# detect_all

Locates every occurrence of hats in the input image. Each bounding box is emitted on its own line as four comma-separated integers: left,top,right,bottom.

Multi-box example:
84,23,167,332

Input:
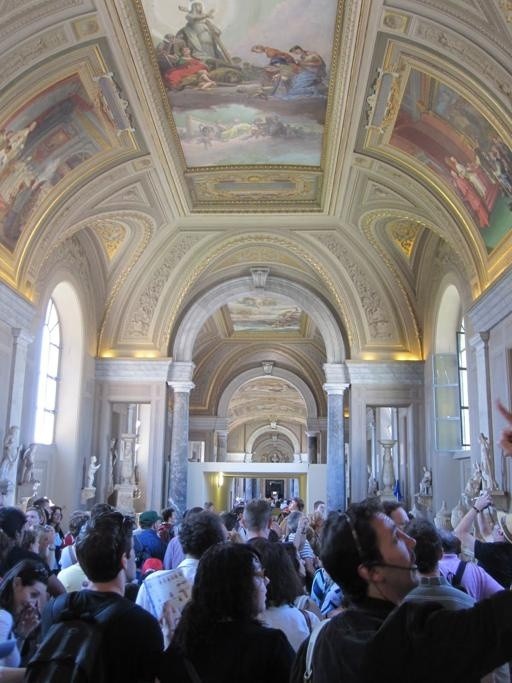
6,547,51,585
139,511,161,525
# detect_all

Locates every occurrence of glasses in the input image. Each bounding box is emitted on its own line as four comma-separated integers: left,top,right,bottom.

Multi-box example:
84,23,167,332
244,567,266,579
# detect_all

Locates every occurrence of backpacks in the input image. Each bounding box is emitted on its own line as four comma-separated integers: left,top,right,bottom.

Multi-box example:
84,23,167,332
23,591,129,683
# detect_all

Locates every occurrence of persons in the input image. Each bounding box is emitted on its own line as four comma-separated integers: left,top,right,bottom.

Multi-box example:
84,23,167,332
465,432,499,492
2,493,512,683
0,119,39,166
122,442,135,484
446,134,511,225
21,443,37,484
419,465,432,496
158,2,327,98
0,425,22,494
88,456,101,488
